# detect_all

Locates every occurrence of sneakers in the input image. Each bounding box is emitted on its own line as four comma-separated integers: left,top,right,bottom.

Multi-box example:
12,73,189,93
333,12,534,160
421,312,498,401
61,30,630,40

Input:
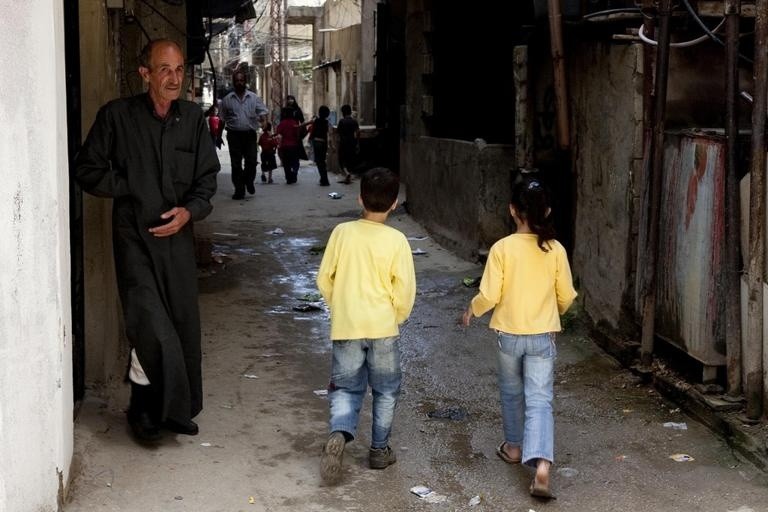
269,178,273,183
287,172,297,183
345,174,352,184
320,433,345,485
262,175,267,181
320,178,330,185
369,444,397,468
245,177,255,194
232,192,244,200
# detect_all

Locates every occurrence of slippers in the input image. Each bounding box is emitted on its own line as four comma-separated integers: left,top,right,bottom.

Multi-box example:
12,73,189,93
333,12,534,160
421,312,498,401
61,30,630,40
530,478,551,497
497,441,522,463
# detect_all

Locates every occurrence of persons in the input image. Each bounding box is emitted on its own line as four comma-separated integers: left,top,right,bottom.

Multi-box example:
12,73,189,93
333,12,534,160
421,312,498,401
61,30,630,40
208,106,220,147
280,95,308,168
276,108,304,185
70,37,220,446
461,177,579,500
293,105,333,187
329,105,362,184
314,165,416,487
214,71,270,201
258,123,279,185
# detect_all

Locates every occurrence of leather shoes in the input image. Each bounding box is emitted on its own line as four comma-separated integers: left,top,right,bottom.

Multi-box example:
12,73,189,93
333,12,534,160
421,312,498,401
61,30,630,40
169,418,198,435
128,407,166,444
